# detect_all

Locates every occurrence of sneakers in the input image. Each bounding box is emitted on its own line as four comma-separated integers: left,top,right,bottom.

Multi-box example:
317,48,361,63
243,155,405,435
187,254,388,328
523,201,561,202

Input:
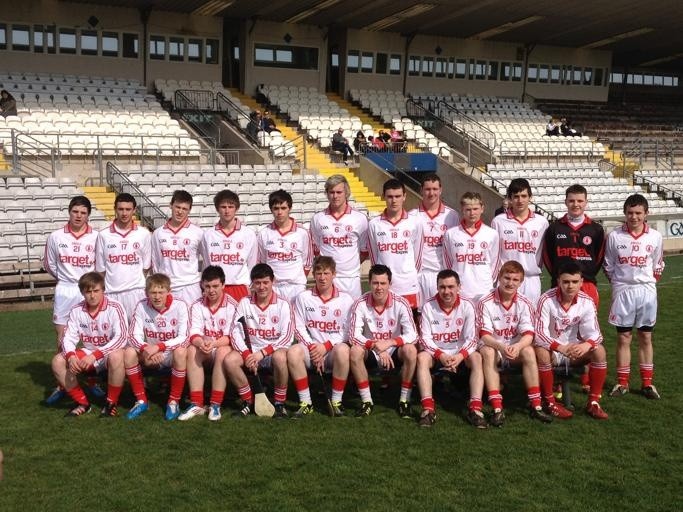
354,402,374,417
274,403,287,420
396,401,416,419
608,384,631,397
231,402,253,418
126,403,149,419
166,404,180,421
333,401,347,418
641,385,662,401
291,403,314,419
177,403,222,422
45,383,119,418
419,378,611,430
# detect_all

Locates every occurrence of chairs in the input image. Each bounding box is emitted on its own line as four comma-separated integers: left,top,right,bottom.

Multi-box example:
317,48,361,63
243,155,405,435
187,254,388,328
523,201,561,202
0,69,683,303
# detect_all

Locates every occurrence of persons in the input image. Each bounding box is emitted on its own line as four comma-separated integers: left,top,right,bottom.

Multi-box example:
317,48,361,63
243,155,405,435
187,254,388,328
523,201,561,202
310,173,371,306
50,271,129,421
152,190,205,301
244,106,410,164
601,194,665,399
532,257,612,420
344,264,420,423
95,194,155,323
416,268,490,429
561,117,584,138
538,183,606,399
440,191,503,304
0,91,18,118
178,265,240,423
494,198,510,216
489,178,550,312
123,273,190,421
200,189,258,296
286,256,353,420
406,171,462,310
544,116,560,137
43,194,108,407
367,177,422,323
478,258,553,427
223,263,295,420
255,189,314,300
